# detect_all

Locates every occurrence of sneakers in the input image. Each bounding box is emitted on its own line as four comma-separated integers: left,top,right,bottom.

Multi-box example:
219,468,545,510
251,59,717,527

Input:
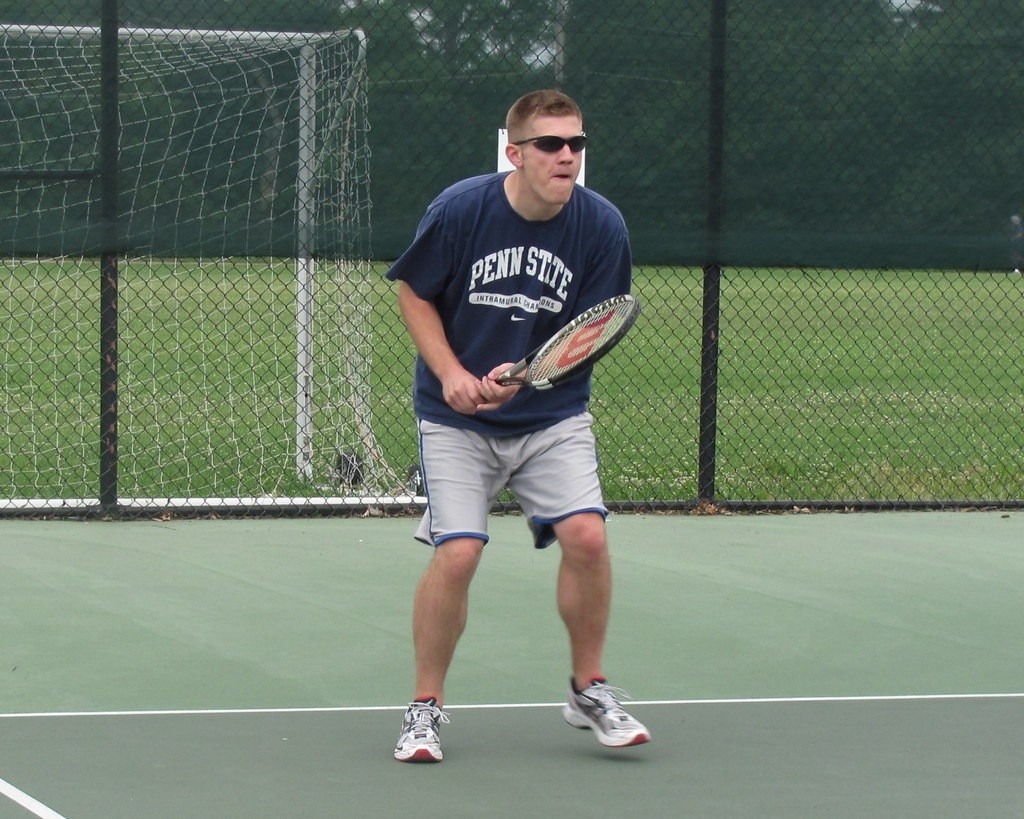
561,672,652,748
393,696,451,764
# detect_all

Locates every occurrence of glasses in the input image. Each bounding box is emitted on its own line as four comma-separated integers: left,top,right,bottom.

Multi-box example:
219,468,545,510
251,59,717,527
513,134,588,152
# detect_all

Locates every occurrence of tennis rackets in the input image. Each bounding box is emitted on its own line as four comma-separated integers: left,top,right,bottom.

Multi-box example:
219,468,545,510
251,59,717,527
468,293,640,393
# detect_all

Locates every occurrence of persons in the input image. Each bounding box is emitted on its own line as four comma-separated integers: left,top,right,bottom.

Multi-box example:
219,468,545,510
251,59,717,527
386,88,652,763
